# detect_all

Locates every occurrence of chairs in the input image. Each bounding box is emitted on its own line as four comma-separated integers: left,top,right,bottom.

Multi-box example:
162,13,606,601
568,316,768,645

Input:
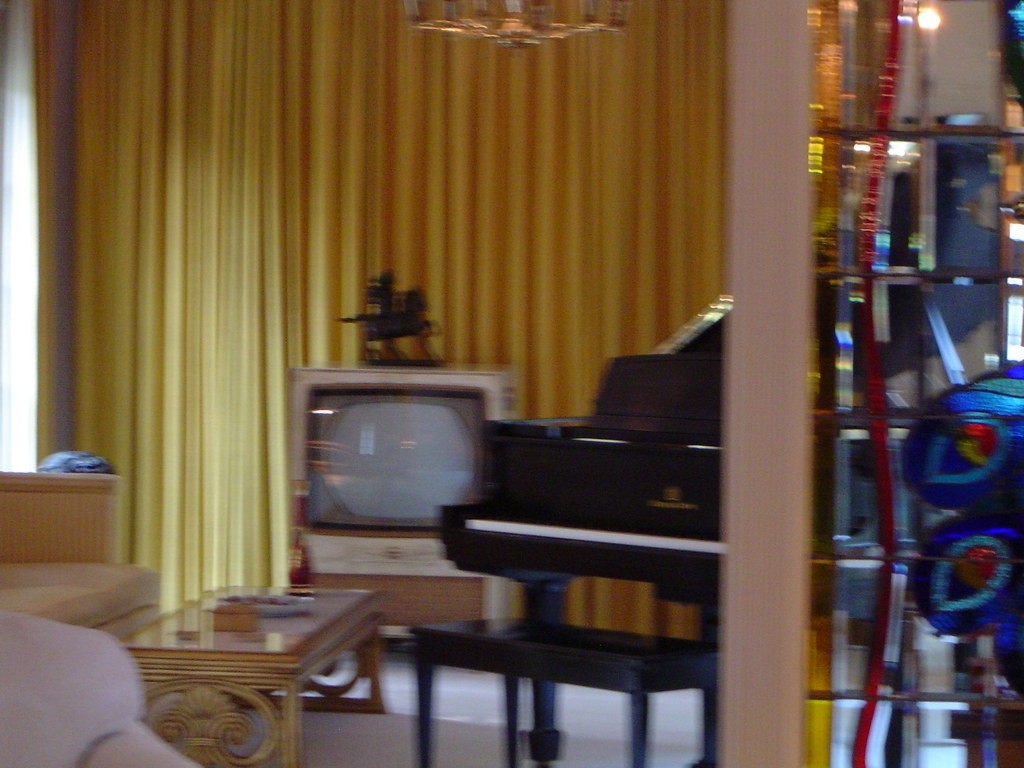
0,610,200,768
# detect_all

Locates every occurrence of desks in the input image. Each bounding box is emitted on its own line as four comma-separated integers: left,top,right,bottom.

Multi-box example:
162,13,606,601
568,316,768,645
113,589,386,768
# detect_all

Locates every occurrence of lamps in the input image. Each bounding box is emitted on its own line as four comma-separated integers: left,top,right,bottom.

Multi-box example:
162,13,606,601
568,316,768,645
404,0,631,50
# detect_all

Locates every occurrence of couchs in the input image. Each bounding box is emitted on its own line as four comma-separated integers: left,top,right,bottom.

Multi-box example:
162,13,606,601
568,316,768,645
0,471,159,637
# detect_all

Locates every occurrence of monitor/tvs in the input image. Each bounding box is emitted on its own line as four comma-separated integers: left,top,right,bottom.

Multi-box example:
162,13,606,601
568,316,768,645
285,366,512,578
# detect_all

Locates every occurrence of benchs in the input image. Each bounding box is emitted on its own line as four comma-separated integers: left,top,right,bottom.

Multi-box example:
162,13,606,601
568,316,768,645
405,616,716,768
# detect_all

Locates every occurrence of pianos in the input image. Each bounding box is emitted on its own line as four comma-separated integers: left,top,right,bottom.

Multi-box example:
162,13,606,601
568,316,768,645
436,350,721,768
